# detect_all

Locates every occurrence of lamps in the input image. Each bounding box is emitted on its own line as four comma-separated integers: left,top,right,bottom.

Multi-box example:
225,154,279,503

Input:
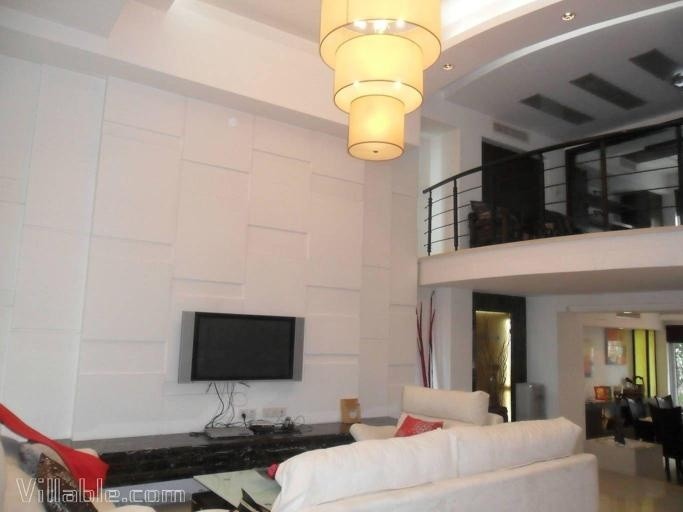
318,0,443,160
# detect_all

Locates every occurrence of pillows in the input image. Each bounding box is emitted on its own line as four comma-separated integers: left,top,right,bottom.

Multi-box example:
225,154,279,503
34,451,98,512
18,438,68,475
394,413,444,437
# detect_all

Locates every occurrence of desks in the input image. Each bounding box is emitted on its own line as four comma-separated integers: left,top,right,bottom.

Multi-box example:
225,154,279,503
76,416,400,490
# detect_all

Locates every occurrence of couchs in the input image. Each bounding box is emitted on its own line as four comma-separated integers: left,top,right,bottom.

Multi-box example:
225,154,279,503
192,414,602,512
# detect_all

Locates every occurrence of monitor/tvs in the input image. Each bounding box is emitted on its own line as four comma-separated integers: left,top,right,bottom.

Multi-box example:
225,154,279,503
176,311,305,384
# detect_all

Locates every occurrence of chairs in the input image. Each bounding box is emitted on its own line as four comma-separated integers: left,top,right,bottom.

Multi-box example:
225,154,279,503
624,395,683,482
1,400,162,512
346,383,503,442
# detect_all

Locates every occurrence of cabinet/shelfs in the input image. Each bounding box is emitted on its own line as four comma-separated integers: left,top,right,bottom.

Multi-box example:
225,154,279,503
586,400,617,439
